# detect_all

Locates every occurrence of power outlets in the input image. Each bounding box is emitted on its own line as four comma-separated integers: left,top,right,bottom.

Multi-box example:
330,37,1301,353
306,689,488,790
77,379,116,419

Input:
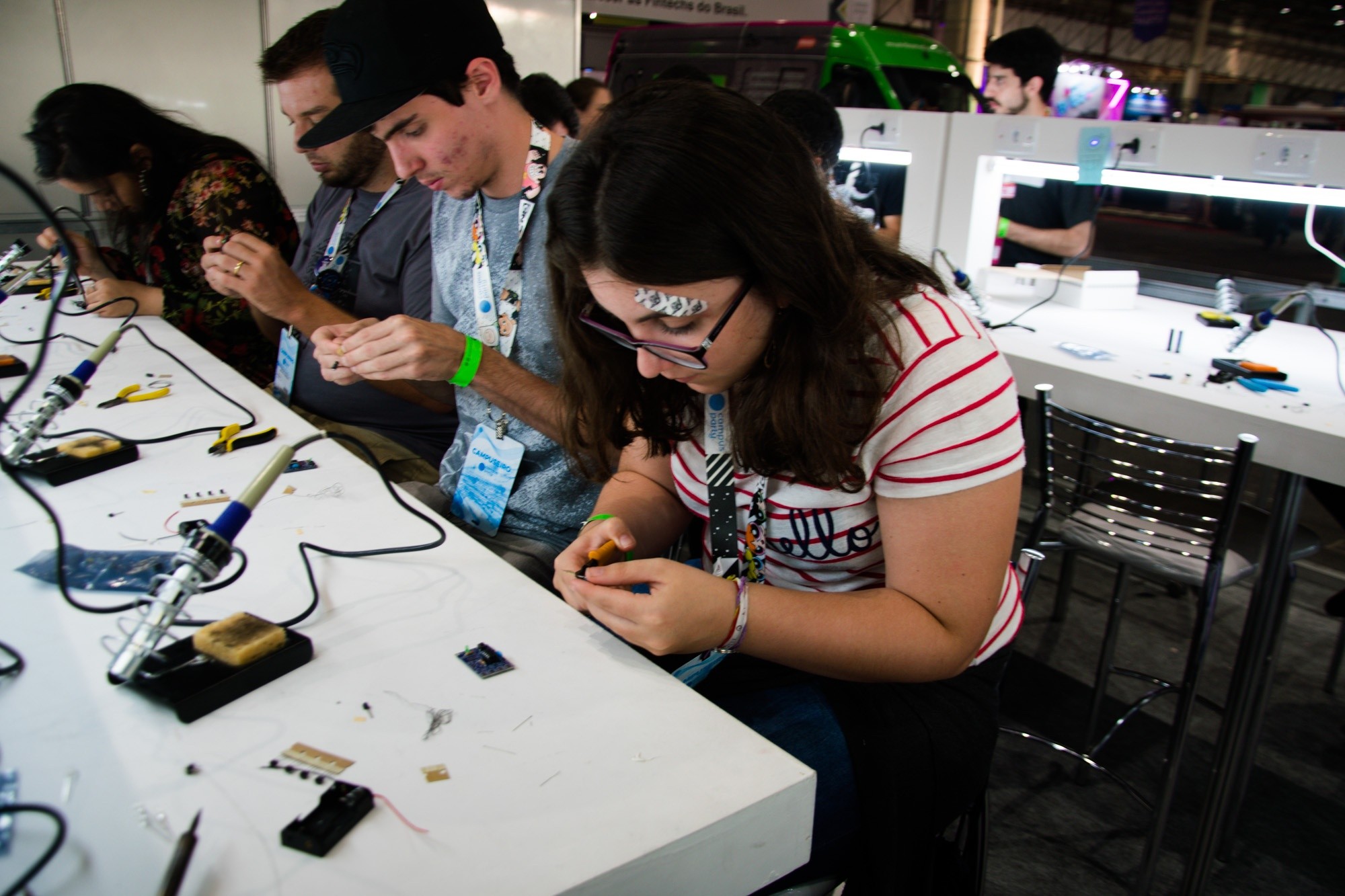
1111,129,1159,166
869,119,895,145
1255,132,1317,175
993,122,1036,154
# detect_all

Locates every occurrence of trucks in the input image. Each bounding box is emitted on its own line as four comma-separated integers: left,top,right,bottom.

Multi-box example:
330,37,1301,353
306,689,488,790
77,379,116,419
601,16,997,114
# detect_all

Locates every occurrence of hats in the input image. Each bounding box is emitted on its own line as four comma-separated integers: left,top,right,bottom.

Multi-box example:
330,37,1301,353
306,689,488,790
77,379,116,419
296,0,504,149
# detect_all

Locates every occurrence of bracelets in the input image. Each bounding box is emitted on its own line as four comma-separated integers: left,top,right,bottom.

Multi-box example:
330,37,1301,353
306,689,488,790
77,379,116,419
713,575,750,654
577,514,635,562
997,217,1009,239
447,333,483,390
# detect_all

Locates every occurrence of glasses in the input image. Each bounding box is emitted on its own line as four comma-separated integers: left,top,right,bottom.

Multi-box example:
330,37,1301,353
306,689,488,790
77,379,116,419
575,277,754,370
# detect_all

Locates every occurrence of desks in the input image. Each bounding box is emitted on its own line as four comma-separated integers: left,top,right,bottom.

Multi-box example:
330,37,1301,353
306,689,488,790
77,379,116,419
934,273,1345,798
0,256,817,896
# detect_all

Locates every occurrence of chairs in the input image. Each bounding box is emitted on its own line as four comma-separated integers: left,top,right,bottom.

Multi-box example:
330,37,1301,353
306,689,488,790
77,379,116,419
606,322,1343,896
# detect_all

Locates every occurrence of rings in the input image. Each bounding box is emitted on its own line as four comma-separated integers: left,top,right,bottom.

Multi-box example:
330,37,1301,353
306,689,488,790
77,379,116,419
234,261,245,276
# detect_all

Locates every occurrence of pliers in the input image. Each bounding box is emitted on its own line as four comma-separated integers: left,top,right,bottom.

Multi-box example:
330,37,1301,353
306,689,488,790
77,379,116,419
34,283,79,300
97,384,170,409
1234,375,1299,393
208,423,278,454
574,540,617,580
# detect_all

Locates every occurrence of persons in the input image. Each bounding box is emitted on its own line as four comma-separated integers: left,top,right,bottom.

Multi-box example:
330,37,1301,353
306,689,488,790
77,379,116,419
823,70,902,239
21,83,301,389
523,74,612,141
1303,476,1345,617
983,24,1099,267
546,81,1028,895
759,89,844,186
296,0,628,589
201,10,459,484
